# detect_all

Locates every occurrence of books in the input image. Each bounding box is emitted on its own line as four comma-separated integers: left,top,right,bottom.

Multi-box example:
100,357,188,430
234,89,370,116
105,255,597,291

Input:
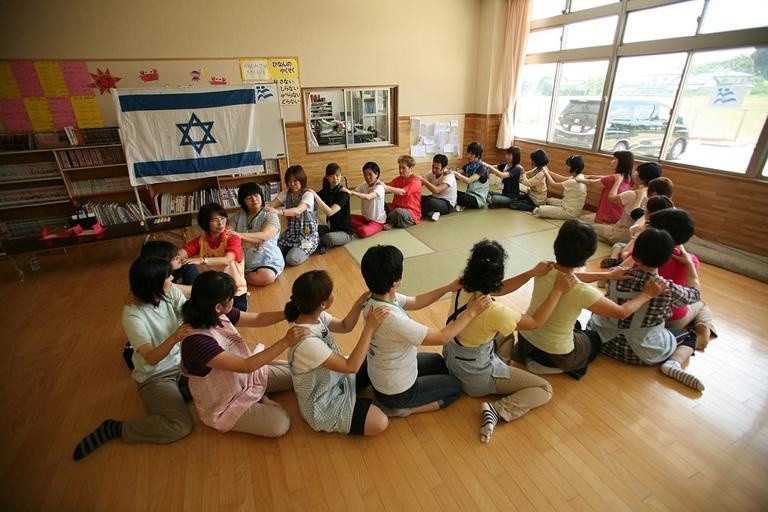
78,200,153,226
0,126,122,152
0,162,62,181
0,213,76,238
220,187,241,208
57,147,127,169
71,177,135,195
257,182,281,203
232,159,278,178
154,192,222,215
0,185,70,206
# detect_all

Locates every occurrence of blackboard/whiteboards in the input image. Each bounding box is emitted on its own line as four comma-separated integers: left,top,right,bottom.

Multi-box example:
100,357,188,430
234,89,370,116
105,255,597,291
1,56,304,130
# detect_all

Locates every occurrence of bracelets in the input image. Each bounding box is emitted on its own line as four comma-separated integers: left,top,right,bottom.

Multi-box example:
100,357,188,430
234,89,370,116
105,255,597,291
281,209,285,216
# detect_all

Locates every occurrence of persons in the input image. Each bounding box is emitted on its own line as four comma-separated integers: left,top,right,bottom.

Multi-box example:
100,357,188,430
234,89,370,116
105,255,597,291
630,196,673,236
479,146,522,207
446,142,488,212
303,163,354,254
261,164,321,265
511,219,669,381
73,256,194,461
586,228,705,391
621,209,717,351
443,239,577,443
575,152,639,228
123,240,202,368
338,162,388,238
179,202,248,310
378,155,428,230
532,155,587,219
591,162,661,246
509,149,549,212
226,182,285,285
180,270,312,437
601,177,680,268
362,245,492,417
415,154,458,221
284,272,391,437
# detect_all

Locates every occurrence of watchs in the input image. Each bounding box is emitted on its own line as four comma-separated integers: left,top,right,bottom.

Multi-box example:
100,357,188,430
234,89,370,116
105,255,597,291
202,257,207,264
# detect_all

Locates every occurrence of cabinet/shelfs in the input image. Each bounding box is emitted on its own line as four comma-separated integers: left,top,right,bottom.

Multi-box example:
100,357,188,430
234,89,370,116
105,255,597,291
0,143,288,254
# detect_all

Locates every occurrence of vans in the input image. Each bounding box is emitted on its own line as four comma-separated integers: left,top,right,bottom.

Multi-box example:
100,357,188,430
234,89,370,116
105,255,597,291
554,99,688,160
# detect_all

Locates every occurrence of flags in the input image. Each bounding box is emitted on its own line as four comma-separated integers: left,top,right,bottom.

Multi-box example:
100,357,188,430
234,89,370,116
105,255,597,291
110,85,265,186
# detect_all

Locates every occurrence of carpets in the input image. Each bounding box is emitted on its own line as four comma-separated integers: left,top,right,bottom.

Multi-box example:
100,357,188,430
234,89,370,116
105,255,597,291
316,175,613,330
